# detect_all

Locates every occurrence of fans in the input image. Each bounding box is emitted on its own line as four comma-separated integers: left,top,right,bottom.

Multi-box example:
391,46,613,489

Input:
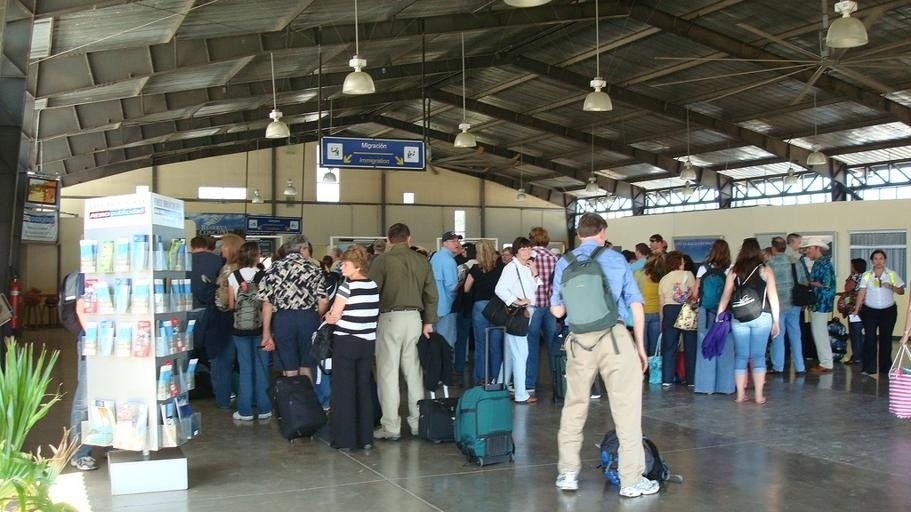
645,1,911,107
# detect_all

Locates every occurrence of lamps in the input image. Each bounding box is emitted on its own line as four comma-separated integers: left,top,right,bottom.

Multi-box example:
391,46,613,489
805,85,827,166
453,32,477,147
582,1,613,112
265,52,291,139
824,0,869,49
783,160,798,185
252,146,600,204
341,1,375,94
682,178,694,195
679,103,698,182
503,1,551,8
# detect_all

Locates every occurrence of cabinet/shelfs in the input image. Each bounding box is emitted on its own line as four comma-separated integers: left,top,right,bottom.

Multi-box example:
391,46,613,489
77,184,207,497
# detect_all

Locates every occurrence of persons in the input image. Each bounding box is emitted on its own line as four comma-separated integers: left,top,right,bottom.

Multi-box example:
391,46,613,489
187,229,388,453
843,258,866,365
618,232,836,404
899,307,910,345
851,250,905,375
58,270,118,471
549,214,659,497
411,227,603,404
366,222,439,440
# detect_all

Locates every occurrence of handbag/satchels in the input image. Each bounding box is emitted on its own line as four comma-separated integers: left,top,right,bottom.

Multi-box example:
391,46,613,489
310,324,333,361
888,344,911,418
674,301,699,331
649,333,663,384
837,290,856,313
482,297,529,336
792,283,818,306
827,318,847,361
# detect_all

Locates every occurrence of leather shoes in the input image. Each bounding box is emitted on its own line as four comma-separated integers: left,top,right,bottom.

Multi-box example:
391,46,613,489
768,365,833,376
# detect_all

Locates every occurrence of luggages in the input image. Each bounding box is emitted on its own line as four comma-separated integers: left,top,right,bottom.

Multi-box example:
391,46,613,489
454,326,513,466
257,345,328,443
419,398,456,443
553,355,566,404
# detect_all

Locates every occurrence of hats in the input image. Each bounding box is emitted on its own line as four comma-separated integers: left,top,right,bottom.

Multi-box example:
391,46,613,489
442,232,463,242
799,237,830,251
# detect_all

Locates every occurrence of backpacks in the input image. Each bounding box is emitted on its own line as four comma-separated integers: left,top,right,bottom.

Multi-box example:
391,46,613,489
560,247,618,334
233,269,266,336
57,272,82,335
600,430,671,486
731,264,767,322
699,263,729,312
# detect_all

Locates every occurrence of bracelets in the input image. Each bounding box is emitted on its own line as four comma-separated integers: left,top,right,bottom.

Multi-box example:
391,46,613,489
809,282,811,286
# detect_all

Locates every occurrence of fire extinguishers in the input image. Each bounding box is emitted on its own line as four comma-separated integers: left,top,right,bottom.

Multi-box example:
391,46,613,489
8,266,22,329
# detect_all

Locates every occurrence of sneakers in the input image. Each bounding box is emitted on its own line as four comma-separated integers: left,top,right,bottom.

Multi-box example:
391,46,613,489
556,471,579,490
232,412,254,421
619,478,661,497
412,430,419,437
373,427,401,441
509,382,538,403
103,450,107,458
258,412,272,419
71,455,98,470
